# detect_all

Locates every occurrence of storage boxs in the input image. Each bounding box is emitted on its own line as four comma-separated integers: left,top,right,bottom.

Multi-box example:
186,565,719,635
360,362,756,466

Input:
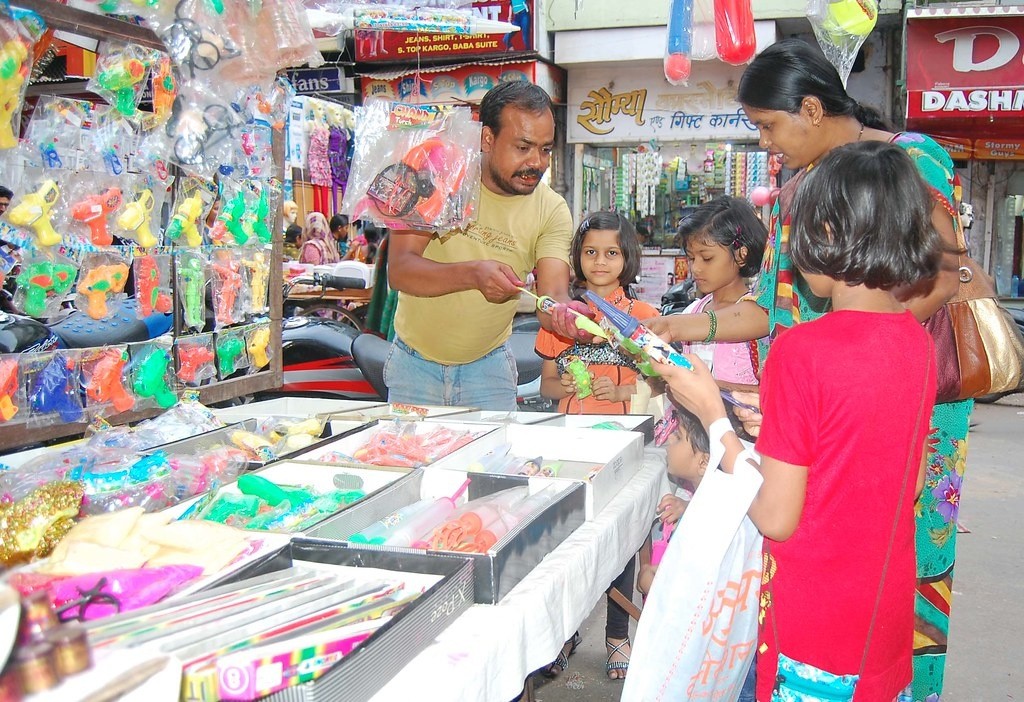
282,261,375,289
0,395,654,701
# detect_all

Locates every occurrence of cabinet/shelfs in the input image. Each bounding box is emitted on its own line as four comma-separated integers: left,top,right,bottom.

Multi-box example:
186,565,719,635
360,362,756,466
582,146,769,249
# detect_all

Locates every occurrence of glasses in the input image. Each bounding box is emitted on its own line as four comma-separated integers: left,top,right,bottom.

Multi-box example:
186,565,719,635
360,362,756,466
175,104,244,166
163,17,220,81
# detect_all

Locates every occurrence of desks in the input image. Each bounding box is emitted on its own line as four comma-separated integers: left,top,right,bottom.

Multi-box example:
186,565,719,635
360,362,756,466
360,443,669,702
283,286,374,321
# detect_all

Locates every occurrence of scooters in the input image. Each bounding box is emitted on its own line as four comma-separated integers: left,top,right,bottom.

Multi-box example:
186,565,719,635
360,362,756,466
660,278,702,316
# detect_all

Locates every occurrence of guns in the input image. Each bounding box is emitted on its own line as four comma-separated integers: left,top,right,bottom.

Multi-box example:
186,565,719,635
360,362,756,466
0,31,286,427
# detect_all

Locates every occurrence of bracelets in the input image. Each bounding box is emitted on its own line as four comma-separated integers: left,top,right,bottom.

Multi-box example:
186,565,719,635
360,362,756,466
702,310,716,344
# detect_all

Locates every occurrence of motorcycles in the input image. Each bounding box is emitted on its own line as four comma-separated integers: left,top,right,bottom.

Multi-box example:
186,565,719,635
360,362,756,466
209,315,553,401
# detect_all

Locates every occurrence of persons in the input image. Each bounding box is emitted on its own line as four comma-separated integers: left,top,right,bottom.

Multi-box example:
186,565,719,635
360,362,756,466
382,80,596,411
533,38,974,702
283,200,391,318
307,99,360,218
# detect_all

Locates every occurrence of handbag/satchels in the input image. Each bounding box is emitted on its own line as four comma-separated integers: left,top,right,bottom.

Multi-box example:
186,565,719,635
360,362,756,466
619,416,765,702
888,131,1024,405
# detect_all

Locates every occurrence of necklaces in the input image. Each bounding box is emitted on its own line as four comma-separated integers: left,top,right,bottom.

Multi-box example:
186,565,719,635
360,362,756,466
858,122,864,141
627,299,635,316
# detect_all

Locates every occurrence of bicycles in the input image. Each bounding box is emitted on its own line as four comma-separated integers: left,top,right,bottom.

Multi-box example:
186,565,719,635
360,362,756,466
283,273,365,330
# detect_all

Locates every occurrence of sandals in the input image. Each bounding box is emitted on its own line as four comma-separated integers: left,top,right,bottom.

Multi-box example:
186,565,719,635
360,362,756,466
605,635,631,679
541,630,582,679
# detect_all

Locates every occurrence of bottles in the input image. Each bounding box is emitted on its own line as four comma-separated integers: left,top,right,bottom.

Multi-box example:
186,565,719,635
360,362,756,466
1010,275,1019,298
